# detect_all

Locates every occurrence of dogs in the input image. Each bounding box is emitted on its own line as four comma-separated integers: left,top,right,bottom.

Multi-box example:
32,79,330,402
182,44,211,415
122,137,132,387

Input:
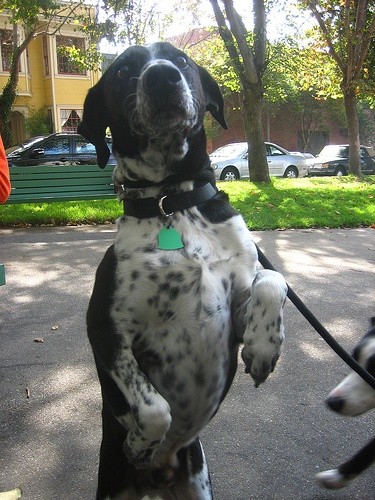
76,41,288,500
313,315,374,491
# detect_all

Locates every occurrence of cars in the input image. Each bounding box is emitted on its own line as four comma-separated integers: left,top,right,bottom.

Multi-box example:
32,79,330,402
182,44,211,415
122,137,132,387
5,132,117,167
303,153,317,168
208,141,307,181
305,144,375,176
